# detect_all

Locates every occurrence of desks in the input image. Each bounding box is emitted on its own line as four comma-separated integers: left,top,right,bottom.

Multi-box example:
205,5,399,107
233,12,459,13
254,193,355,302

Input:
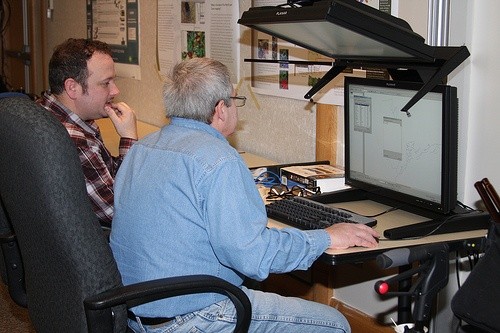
95,118,488,333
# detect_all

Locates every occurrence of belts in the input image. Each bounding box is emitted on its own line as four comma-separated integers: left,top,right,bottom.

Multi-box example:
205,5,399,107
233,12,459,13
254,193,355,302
127,310,175,326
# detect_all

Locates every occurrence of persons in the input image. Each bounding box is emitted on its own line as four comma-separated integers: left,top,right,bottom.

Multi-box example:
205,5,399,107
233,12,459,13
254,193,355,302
34,37,137,223
113,57,380,333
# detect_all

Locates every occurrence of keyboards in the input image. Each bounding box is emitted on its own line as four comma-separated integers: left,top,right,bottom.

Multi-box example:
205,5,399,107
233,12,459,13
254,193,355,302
264,196,377,231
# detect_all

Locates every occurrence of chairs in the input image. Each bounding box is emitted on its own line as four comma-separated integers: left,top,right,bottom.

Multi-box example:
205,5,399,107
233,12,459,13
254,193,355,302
0,93,252,333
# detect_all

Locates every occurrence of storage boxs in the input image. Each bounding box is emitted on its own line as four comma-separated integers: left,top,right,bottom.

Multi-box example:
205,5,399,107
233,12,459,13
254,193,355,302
281,164,351,194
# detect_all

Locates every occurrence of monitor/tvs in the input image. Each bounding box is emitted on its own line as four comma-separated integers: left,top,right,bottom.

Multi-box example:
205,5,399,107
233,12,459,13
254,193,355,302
342,76,458,214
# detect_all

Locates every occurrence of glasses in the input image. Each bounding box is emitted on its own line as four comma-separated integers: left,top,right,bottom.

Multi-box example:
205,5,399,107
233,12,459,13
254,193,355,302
266,183,321,199
215,96,247,108
